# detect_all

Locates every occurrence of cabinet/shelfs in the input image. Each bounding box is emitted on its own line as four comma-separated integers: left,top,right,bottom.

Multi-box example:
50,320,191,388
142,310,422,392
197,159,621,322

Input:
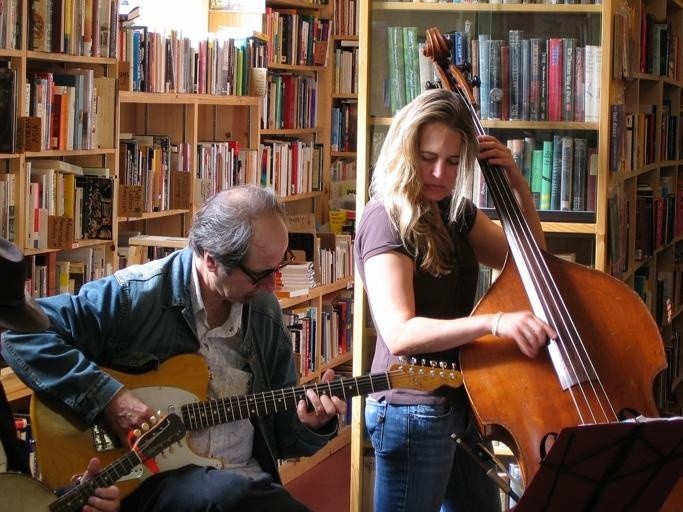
359,0,681,465
0,1,359,501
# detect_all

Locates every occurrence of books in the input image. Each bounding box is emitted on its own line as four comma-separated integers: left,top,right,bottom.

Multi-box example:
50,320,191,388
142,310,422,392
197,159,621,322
461,129,597,212
261,69,318,130
1,2,119,58
197,140,258,201
607,178,682,413
386,26,601,124
24,242,111,298
330,104,356,193
334,2,359,95
274,208,353,298
265,8,331,68
118,131,190,213
613,2,681,174
282,289,352,377
260,136,324,198
1,60,121,154
1,157,114,253
118,230,178,271
117,4,267,96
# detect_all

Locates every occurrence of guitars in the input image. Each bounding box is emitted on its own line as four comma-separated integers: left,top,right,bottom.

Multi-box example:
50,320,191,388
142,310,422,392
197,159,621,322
31,353,464,500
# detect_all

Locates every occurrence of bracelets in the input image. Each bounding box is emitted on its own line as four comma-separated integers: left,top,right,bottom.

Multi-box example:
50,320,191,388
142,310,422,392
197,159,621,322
491,310,504,337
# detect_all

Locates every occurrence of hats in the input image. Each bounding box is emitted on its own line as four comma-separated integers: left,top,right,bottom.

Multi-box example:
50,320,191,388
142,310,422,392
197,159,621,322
0,234,52,333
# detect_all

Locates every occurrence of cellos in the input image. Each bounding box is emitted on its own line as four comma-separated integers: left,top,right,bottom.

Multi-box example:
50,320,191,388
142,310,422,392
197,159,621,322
420,25,683,510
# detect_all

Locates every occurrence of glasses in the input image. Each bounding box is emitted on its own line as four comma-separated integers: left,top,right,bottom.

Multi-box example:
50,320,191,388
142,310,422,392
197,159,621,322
240,249,295,285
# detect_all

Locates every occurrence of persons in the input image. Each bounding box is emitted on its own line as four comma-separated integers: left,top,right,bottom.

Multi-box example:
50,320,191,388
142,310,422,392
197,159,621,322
0,179,346,511
354,89,556,511
74,456,122,510
